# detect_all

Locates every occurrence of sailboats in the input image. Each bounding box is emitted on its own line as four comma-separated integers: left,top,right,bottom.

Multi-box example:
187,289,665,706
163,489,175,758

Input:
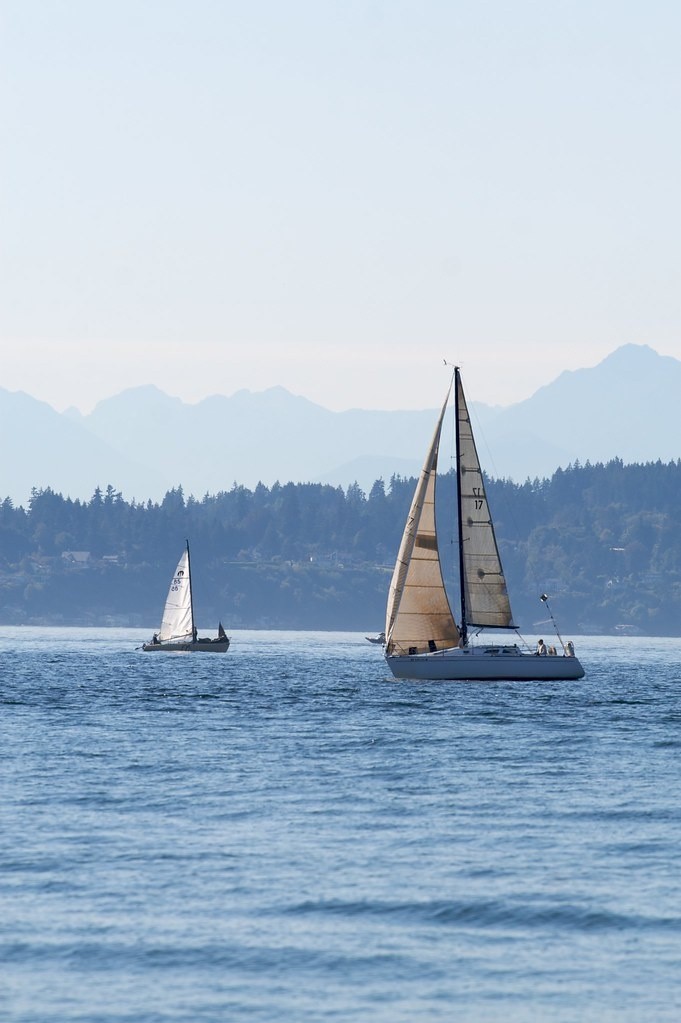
141,541,231,653
379,366,587,680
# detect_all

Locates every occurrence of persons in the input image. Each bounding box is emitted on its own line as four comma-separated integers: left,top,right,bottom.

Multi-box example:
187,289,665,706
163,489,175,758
457,621,468,645
153,634,160,644
534,638,575,657
